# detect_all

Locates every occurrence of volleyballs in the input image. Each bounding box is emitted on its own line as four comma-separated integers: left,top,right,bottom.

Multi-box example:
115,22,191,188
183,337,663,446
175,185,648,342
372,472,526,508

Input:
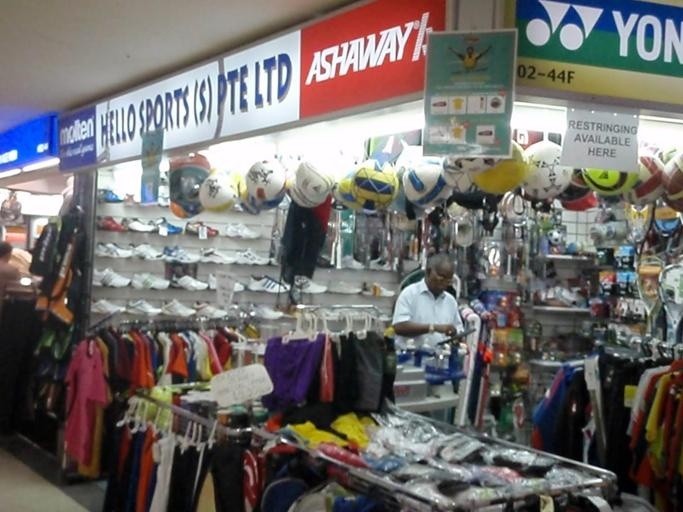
200,140,683,214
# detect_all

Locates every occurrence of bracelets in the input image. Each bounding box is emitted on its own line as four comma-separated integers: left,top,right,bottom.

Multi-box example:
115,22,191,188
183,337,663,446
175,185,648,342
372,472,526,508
428,323,434,333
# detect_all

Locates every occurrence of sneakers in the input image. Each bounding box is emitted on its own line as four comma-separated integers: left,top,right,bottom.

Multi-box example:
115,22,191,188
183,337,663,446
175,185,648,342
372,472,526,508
327,219,353,235
99,189,126,205
95,268,395,322
95,215,261,239
316,251,394,271
96,242,278,265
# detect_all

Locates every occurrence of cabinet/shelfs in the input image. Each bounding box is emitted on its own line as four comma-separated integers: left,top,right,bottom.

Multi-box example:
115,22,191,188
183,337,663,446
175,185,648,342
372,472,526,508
530,252,598,369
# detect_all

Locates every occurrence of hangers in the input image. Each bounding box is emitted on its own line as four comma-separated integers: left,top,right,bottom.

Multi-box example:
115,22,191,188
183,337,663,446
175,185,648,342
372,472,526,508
114,389,223,458
281,303,387,344
83,315,258,355
604,332,683,380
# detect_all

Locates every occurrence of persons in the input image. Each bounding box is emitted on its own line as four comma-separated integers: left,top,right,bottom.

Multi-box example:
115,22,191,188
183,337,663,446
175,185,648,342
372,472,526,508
0,240,23,302
390,251,466,352
